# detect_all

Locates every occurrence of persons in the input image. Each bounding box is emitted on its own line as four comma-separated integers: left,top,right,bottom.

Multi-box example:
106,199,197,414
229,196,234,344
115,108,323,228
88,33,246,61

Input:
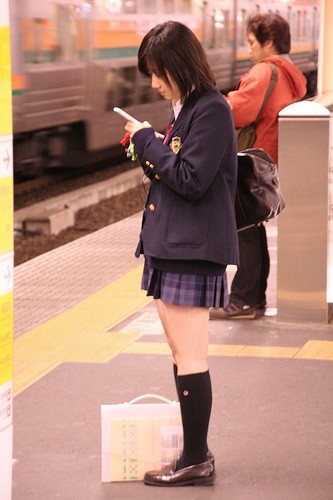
209,14,307,322
125,21,237,488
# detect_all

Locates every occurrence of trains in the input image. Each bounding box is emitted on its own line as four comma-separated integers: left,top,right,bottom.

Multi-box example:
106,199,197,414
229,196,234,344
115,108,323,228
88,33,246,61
10,0,323,179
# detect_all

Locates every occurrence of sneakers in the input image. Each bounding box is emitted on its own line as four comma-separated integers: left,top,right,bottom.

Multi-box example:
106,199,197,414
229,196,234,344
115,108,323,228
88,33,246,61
209,295,266,319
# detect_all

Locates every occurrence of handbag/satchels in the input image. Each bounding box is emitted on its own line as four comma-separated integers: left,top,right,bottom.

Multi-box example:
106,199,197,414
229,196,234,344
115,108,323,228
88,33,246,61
235,60,277,152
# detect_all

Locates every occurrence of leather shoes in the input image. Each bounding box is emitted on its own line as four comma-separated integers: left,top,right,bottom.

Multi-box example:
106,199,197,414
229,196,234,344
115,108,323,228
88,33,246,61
144,450,216,487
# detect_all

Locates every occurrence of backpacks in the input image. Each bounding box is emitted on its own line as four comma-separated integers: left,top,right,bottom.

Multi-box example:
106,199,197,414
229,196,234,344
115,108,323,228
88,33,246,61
236,148,285,233
